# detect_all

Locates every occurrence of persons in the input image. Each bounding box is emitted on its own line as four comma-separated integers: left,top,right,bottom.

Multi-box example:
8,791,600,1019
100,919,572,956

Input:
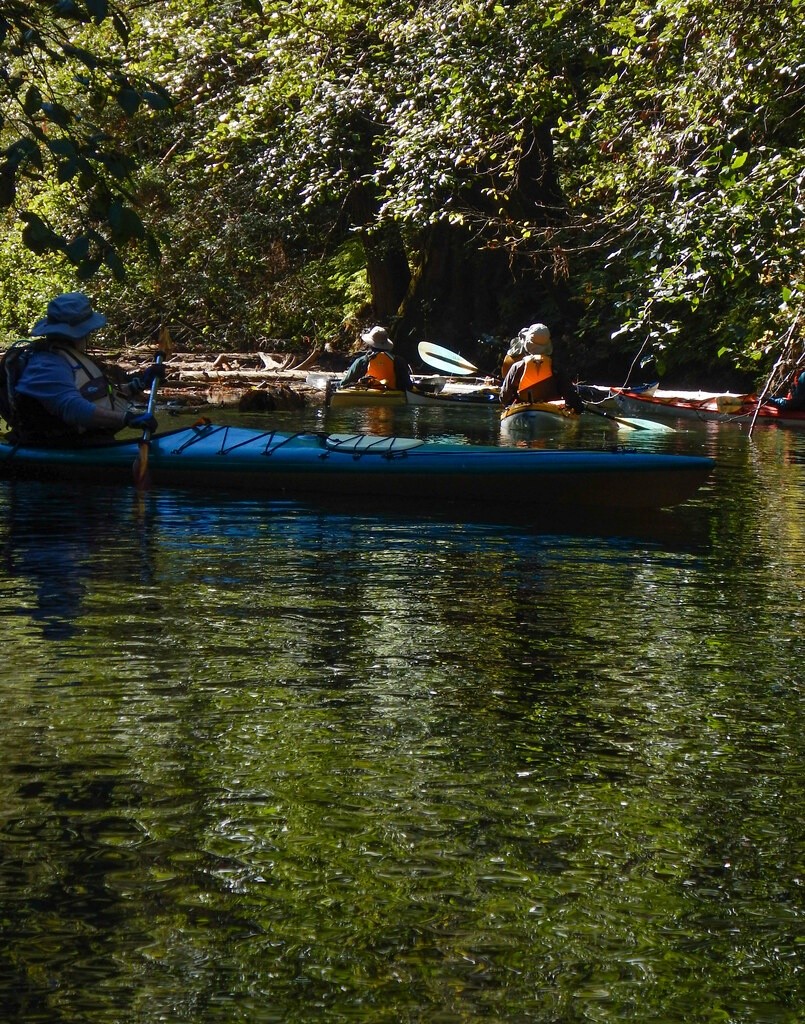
17,292,165,448
335,326,413,392
498,322,584,414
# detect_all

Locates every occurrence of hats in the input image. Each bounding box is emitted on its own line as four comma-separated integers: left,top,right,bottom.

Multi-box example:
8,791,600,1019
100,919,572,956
30,293,106,338
360,325,394,349
517,322,553,356
507,337,523,356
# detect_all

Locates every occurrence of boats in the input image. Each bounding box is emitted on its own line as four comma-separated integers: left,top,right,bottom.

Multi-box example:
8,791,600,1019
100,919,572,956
407,374,505,403
0,419,719,516
609,385,805,427
500,402,581,444
316,383,407,407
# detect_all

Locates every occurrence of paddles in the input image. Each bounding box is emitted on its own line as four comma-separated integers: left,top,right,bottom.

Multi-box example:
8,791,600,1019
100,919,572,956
415,336,670,433
134,324,173,484
305,372,446,394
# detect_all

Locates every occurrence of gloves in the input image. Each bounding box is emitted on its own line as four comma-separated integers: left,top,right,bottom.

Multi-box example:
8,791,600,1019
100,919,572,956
122,411,158,433
132,364,167,392
